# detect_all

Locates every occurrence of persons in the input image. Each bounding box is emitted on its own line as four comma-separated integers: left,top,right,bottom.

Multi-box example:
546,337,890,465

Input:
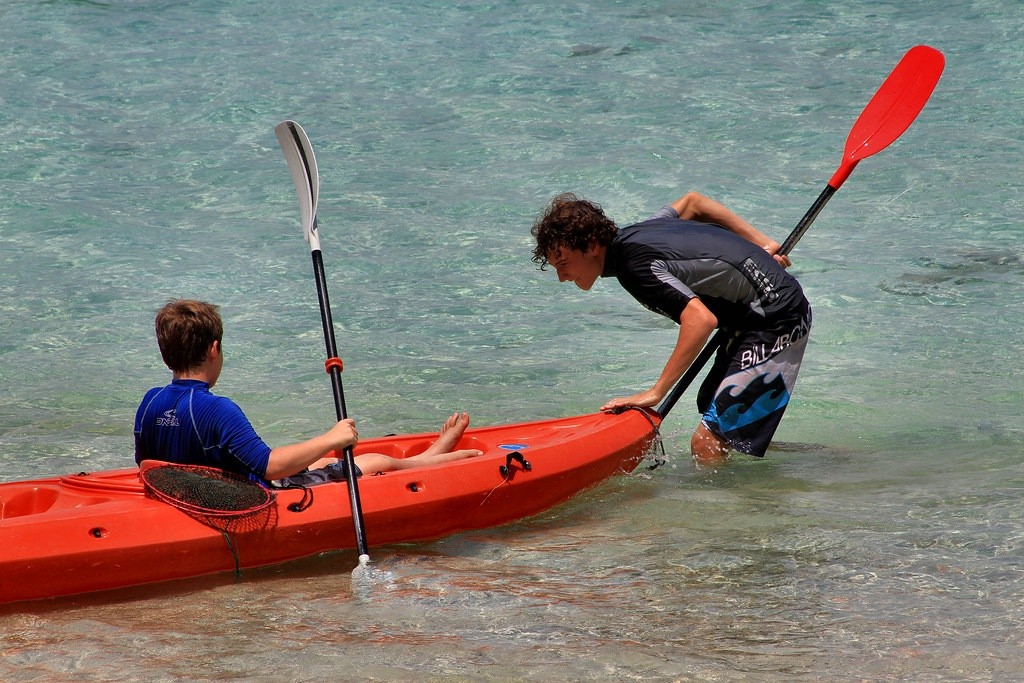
531,191,813,470
134,296,483,489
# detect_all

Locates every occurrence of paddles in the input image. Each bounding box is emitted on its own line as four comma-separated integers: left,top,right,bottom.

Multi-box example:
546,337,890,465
274,119,376,574
656,44,947,422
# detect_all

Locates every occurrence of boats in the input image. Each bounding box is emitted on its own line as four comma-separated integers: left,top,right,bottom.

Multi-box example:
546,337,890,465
1,404,664,610
